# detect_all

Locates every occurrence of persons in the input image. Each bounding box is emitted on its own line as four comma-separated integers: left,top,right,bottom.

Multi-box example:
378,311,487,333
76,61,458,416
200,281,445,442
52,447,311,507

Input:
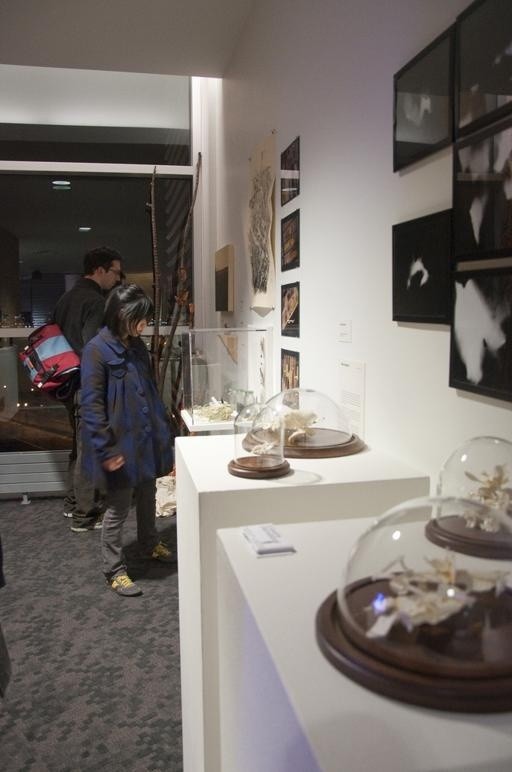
51,247,125,532
81,283,179,597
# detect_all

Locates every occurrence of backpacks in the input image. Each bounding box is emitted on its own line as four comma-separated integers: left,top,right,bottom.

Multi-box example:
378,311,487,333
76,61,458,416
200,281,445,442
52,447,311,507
17,296,77,402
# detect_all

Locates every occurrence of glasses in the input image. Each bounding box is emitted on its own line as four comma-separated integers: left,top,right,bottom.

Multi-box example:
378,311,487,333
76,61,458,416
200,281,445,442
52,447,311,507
107,268,121,275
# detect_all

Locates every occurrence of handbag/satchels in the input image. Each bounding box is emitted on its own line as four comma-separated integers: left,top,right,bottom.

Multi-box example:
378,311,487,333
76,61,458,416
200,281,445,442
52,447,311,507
17,320,81,402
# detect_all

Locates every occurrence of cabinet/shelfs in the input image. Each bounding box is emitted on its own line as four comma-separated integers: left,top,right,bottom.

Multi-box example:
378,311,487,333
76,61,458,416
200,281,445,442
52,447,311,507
173,430,431,772
218,517,512,772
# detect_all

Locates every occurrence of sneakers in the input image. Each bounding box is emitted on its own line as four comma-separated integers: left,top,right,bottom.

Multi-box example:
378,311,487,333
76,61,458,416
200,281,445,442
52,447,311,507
106,569,142,598
142,541,177,563
61,507,79,516
68,518,107,531
